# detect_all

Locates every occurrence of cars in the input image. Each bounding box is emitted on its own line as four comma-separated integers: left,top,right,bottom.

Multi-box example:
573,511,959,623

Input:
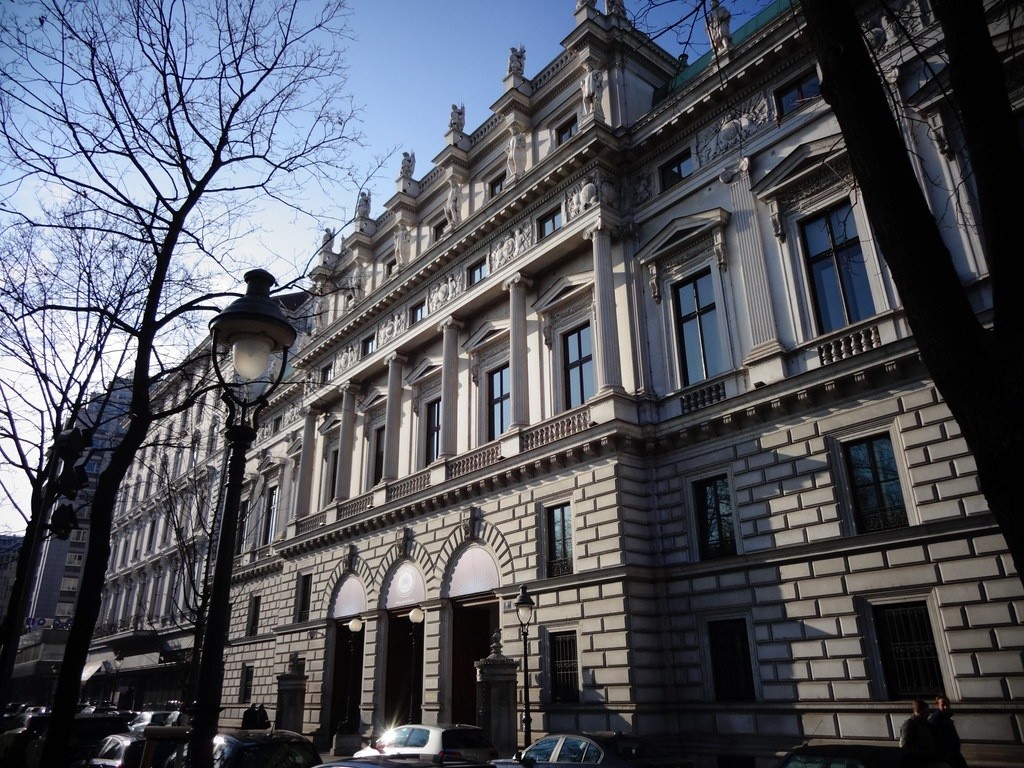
2,700,968,768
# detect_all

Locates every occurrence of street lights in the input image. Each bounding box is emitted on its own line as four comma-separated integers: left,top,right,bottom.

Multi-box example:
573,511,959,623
337,619,363,734
187,269,299,766
409,607,425,724
515,583,535,747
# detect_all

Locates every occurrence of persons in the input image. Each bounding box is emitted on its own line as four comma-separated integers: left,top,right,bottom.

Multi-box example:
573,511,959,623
357,190,371,219
400,148,416,177
898,696,969,768
503,122,527,177
579,63,603,111
448,99,465,132
443,177,464,225
321,227,336,250
351,260,363,298
241,702,272,730
705,0,731,53
394,222,411,265
508,44,527,76
